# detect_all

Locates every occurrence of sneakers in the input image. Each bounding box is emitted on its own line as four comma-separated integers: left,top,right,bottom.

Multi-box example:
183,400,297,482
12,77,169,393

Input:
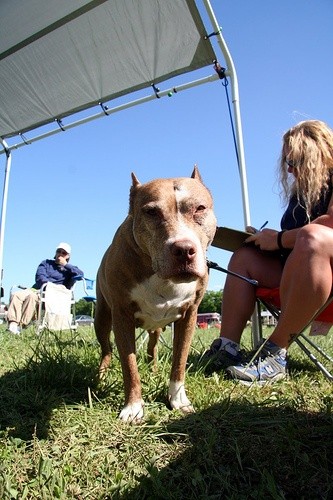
200,338,239,372
226,338,287,382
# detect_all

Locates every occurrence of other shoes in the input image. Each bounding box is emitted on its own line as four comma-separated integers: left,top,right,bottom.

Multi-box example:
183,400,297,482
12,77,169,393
7,323,19,335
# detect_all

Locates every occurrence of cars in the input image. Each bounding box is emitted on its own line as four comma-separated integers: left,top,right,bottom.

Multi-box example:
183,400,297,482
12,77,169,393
72,315,96,325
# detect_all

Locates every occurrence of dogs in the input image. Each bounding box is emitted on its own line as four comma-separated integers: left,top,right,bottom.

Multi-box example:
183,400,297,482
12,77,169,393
91,164,217,423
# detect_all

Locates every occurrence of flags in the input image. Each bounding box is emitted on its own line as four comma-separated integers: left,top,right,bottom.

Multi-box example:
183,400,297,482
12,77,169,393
84,278,93,290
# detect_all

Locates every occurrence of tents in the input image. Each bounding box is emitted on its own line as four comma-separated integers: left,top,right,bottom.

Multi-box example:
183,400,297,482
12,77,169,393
0,0,261,350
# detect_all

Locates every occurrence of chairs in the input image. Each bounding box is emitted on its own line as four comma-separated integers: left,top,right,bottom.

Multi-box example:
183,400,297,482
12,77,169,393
10,277,96,337
255,288,333,380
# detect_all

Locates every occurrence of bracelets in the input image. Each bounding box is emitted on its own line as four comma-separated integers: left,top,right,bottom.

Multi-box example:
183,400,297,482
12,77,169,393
277,229,286,249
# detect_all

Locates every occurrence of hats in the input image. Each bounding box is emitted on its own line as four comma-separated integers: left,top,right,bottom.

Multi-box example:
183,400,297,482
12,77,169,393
57,243,71,253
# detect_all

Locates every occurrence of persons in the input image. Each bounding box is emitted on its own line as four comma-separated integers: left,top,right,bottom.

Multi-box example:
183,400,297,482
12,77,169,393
6,241,84,334
190,123,333,385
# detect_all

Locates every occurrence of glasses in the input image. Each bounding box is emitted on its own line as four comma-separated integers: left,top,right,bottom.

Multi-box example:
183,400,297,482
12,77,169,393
285,158,305,169
57,250,67,255
